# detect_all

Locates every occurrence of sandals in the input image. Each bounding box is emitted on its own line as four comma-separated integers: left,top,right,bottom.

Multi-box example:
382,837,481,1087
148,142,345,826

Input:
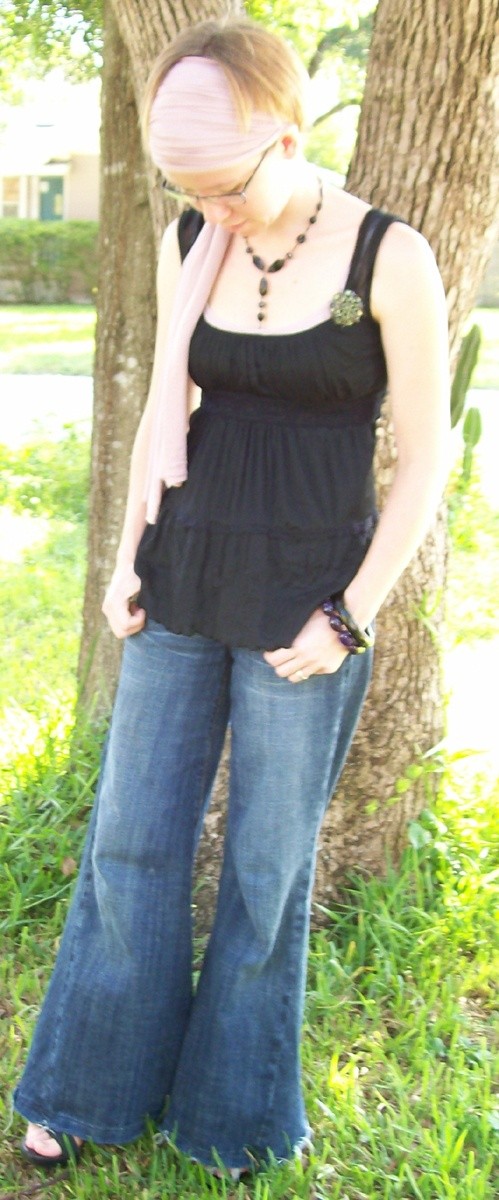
21,1120,89,1161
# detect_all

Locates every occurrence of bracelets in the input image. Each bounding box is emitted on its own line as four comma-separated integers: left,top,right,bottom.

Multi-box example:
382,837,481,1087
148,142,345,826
321,590,376,654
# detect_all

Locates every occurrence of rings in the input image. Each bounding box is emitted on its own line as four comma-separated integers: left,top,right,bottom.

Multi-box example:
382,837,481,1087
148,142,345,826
296,673,308,681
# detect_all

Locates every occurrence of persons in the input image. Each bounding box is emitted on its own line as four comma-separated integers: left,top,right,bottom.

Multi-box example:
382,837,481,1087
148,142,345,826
10,20,453,1193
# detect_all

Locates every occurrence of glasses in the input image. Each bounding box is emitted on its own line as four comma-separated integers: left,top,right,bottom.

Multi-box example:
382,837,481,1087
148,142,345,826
160,138,274,206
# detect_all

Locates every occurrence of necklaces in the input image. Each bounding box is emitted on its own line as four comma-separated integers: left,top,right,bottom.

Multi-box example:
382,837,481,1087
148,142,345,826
241,177,324,321
